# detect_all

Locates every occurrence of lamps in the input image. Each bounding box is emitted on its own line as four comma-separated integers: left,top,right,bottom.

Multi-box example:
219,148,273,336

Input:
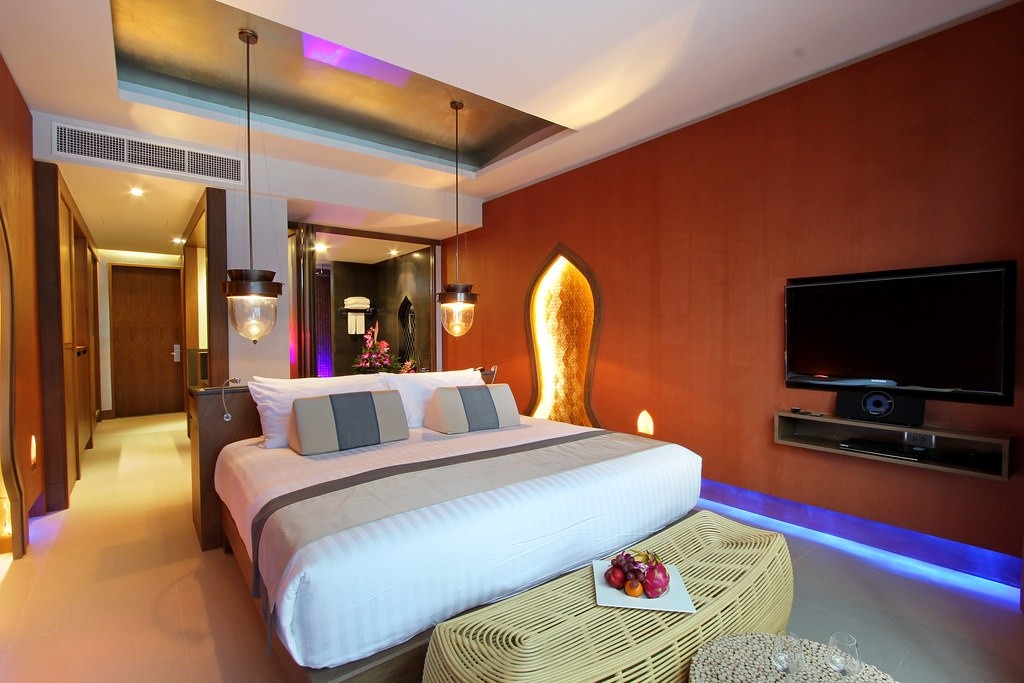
437,100,479,339
223,29,284,345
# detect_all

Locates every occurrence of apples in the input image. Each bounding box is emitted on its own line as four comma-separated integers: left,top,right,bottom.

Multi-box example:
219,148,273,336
605,567,627,590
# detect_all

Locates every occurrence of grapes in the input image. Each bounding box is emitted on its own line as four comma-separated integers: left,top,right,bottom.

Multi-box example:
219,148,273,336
612,550,644,589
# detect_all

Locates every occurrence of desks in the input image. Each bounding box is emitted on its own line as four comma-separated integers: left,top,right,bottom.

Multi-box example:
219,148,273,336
689,631,901,683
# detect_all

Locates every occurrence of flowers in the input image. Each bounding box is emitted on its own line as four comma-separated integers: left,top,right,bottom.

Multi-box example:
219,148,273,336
353,326,401,368
399,353,416,374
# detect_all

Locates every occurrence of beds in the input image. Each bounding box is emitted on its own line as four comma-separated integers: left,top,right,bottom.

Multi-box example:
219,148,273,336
212,415,705,683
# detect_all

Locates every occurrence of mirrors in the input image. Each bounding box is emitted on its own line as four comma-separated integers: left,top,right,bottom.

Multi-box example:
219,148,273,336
298,228,442,378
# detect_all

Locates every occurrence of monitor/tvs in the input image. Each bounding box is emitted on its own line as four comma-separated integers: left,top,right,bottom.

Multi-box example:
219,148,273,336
784,260,1018,406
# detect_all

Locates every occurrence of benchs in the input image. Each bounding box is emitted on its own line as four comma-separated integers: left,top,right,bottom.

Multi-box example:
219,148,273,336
422,511,795,683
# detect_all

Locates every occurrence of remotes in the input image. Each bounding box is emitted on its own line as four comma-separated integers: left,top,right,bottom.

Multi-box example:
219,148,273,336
791,406,801,413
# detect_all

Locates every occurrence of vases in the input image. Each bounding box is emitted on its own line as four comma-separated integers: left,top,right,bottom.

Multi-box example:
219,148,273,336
350,362,403,373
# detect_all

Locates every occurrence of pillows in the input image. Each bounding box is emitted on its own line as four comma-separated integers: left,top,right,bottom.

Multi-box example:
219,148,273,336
249,369,516,455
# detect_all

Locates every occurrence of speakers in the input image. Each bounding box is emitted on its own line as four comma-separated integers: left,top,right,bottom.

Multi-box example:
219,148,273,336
834,392,925,426
